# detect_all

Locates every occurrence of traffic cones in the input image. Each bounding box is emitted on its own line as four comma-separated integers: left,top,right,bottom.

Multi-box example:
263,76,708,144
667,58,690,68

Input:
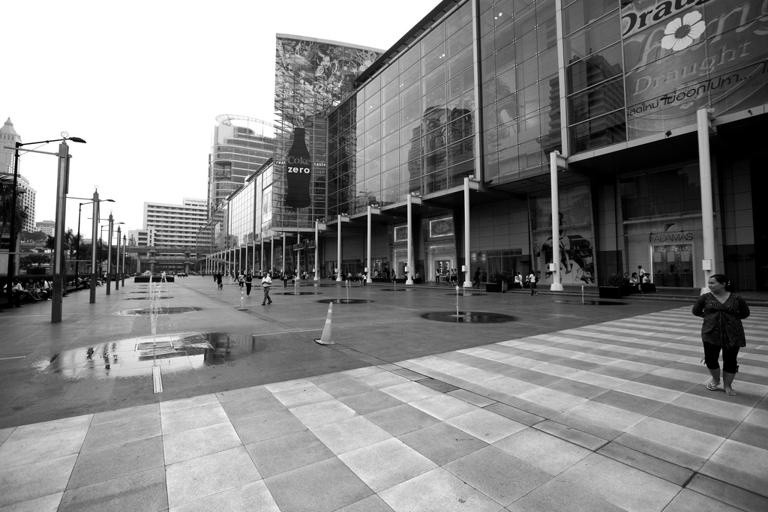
313,301,338,346
237,286,249,311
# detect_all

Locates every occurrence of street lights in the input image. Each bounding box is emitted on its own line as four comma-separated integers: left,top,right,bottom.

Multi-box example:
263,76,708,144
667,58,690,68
100,221,125,278
3,137,88,308
75,198,118,290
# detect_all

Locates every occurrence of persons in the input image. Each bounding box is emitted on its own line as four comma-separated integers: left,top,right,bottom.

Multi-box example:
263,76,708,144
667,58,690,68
234,268,252,296
2,275,54,304
160,270,168,284
609,265,650,292
435,268,459,287
472,267,480,288
415,272,422,284
330,267,395,287
261,271,310,306
214,271,222,288
512,271,536,296
691,273,749,398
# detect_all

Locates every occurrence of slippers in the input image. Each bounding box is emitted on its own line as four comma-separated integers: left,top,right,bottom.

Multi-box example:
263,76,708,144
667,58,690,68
707,383,736,395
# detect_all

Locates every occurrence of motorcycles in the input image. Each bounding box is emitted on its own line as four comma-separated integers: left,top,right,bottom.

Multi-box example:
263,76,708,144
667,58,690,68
3,273,106,306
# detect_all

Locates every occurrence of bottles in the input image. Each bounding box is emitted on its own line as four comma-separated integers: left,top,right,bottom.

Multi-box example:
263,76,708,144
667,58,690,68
284,128,315,209
